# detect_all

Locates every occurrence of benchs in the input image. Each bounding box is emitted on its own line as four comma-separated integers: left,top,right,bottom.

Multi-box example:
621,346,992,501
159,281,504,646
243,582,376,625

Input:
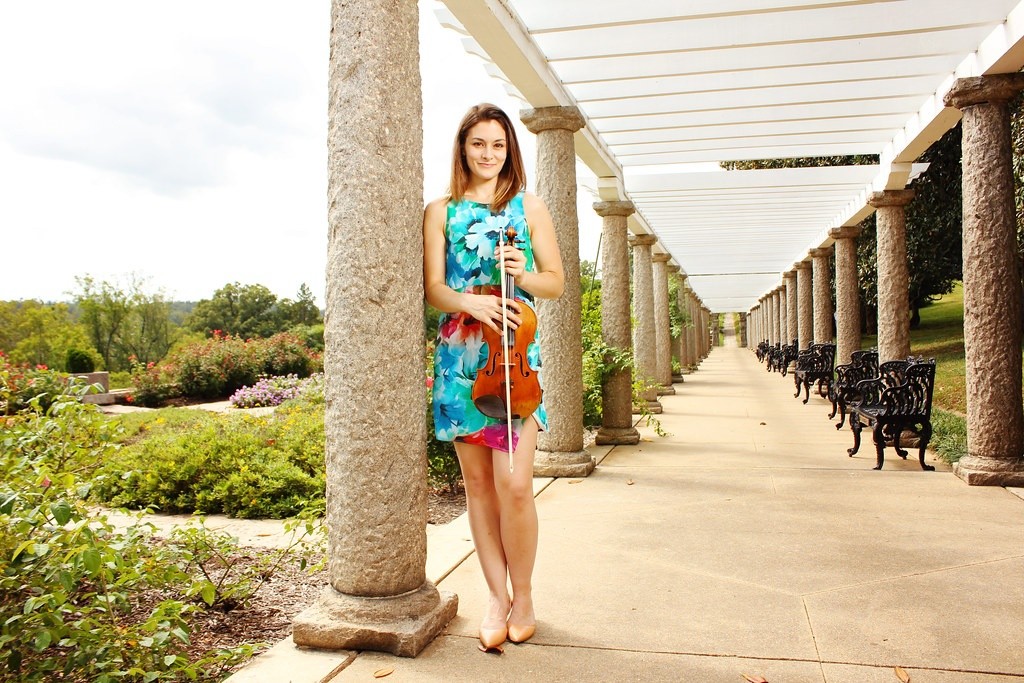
793,344,837,404
828,350,879,430
846,354,937,472
756,337,814,377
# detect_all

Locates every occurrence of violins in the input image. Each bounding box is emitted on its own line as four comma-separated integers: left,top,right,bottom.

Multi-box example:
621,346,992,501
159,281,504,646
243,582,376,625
471,226,541,420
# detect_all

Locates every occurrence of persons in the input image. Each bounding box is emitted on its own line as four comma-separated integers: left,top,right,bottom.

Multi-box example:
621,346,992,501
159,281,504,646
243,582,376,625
420,102,566,649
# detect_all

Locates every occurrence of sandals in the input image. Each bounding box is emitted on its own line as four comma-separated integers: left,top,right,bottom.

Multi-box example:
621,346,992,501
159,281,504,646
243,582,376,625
479,593,512,647
507,621,536,642
